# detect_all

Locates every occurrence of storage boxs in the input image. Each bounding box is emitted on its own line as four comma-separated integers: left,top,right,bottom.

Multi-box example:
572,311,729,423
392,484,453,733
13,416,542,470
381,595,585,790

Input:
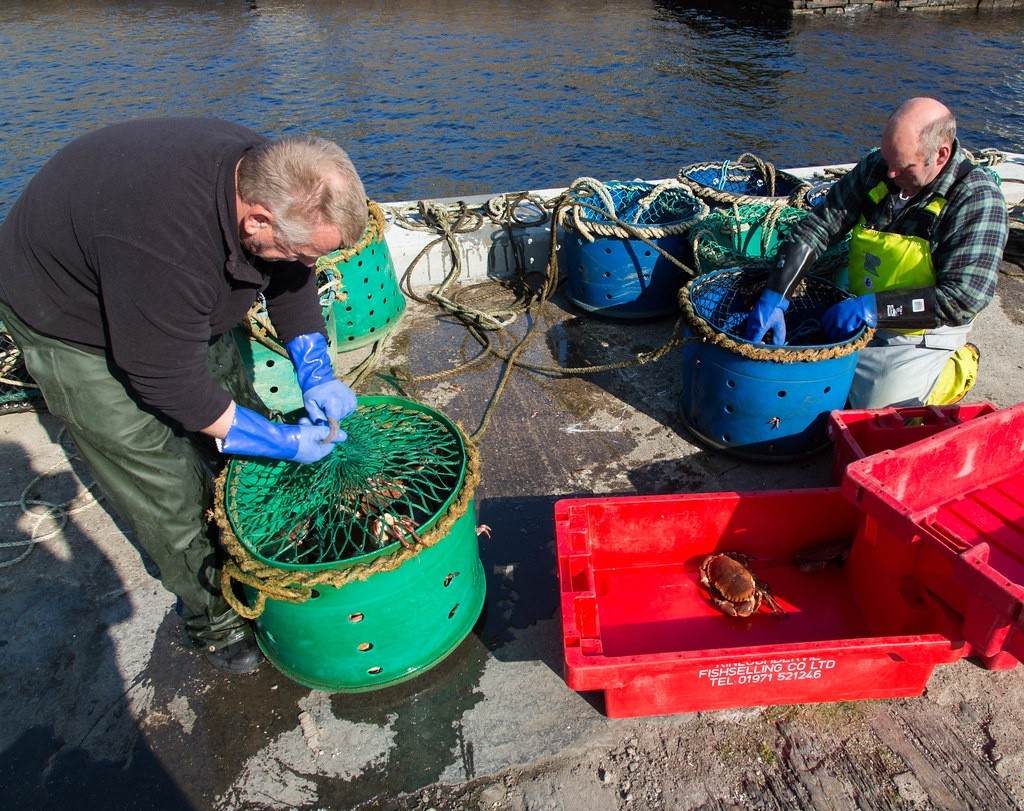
678,264,879,472
244,257,342,418
558,179,711,325
553,484,978,721
313,194,410,353
824,394,1024,673
676,160,807,213
211,392,492,696
685,203,856,294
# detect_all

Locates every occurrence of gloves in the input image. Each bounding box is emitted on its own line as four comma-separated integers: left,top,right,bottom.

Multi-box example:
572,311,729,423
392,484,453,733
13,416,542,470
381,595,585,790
819,285,936,343
744,241,815,346
282,333,356,426
215,403,347,464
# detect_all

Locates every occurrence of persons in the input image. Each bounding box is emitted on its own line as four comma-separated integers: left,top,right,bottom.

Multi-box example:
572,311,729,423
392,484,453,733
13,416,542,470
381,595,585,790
736,94,1008,426
0,120,370,672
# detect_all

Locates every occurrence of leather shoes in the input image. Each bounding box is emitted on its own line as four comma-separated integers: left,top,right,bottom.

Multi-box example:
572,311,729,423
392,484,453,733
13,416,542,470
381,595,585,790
204,635,260,674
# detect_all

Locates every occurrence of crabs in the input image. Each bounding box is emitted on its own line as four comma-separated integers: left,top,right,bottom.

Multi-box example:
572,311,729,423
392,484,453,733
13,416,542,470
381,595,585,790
333,449,436,524
794,540,849,572
371,514,429,551
273,516,311,547
699,551,789,621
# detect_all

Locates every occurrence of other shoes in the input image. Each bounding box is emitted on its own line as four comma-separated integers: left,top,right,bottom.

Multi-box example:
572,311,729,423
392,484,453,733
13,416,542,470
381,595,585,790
946,342,980,405
